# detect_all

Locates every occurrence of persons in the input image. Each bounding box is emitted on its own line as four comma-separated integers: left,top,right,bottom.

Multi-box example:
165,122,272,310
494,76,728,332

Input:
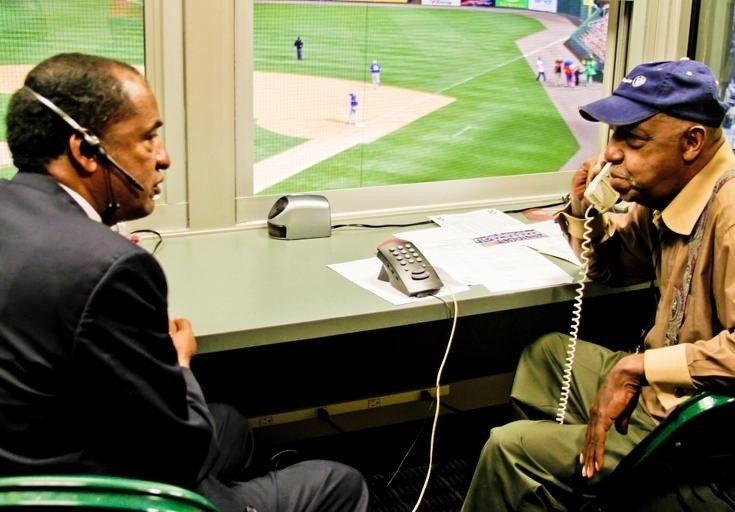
459,57,735,512
536,53,597,87
347,92,359,124
294,36,303,60
369,61,382,88
0,53,370,512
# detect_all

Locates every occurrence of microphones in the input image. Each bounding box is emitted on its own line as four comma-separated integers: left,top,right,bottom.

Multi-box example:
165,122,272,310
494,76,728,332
80,135,145,192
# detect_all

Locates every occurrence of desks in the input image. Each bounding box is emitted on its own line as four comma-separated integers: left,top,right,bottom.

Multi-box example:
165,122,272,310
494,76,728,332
119,194,658,446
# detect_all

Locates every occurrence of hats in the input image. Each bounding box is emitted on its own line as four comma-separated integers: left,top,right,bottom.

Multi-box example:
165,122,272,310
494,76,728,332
579,61,729,129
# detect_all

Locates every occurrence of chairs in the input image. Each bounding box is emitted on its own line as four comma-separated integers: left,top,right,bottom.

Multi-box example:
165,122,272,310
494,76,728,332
514,392,734,511
0,474,222,512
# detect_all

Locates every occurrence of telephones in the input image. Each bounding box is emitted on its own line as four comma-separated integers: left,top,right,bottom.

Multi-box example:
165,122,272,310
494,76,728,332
582,160,621,216
